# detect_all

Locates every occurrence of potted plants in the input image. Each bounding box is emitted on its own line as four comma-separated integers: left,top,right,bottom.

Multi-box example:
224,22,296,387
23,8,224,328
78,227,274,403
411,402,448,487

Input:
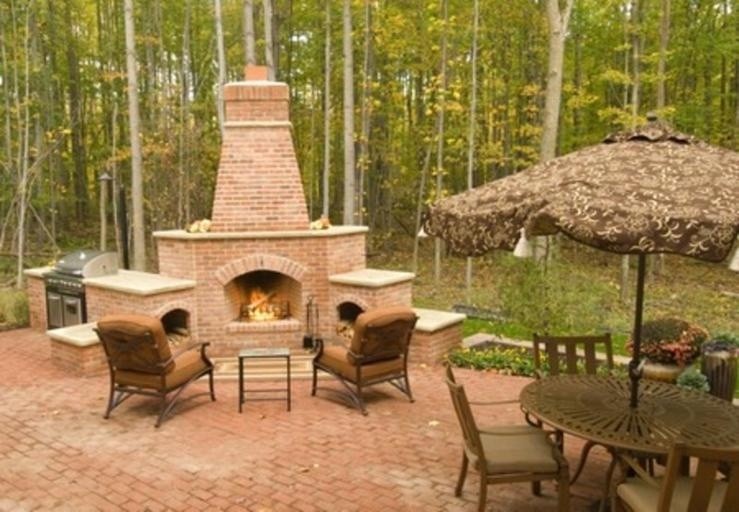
627,318,711,382
702,334,739,403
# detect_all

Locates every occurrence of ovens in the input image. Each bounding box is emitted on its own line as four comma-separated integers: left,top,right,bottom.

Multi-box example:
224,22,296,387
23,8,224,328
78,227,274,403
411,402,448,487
44,274,87,330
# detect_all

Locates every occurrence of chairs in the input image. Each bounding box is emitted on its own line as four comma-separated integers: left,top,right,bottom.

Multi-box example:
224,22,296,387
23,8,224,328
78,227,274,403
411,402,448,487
443,365,570,512
532,332,614,457
311,305,420,416
616,442,739,512
93,315,216,427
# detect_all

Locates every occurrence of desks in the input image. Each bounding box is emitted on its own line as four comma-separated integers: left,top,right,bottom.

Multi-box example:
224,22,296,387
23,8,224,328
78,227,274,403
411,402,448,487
519,373,739,512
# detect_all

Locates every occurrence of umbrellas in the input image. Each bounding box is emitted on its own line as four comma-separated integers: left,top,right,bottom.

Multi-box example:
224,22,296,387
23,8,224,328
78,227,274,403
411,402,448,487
416,115,739,409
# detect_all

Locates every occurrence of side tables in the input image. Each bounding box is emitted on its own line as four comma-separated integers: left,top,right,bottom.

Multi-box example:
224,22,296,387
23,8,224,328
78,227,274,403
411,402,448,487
237,347,291,413
